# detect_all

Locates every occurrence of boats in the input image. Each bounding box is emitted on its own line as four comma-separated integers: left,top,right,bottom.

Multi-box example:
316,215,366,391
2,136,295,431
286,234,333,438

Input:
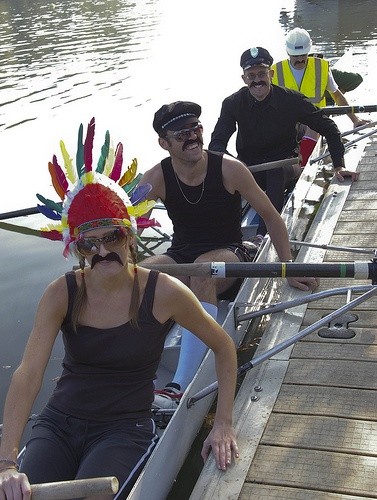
17,106,333,500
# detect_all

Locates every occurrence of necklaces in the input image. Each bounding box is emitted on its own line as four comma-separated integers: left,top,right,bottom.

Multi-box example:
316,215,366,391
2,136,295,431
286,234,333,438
175,174,204,204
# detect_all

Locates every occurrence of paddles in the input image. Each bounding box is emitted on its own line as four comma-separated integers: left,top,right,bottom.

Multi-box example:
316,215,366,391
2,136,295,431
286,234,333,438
320,105,377,116
30,476,119,500
0,156,299,221
73,256,377,286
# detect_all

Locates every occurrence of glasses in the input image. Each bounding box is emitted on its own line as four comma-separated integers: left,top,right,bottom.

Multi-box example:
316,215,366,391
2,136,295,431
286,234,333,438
75,229,129,255
165,125,204,141
243,70,271,80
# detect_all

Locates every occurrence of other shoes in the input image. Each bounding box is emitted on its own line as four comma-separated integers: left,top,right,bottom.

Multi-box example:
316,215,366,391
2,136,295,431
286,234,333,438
242,235,263,249
154,382,183,411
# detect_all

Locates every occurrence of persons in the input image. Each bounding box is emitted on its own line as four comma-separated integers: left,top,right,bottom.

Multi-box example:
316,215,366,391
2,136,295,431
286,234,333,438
126,101,320,412
0,226,240,500
269,27,368,169
208,47,359,242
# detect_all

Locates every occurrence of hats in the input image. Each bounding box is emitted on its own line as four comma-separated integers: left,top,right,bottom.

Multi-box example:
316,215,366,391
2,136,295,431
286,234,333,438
58,170,138,243
239,47,274,69
152,101,201,135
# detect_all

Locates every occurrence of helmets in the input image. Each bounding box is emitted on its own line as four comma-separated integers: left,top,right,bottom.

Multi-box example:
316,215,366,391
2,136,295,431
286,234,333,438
286,27,312,56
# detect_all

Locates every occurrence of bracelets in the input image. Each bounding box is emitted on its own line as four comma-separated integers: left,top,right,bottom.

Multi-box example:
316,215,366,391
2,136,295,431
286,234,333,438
0,460,19,471
